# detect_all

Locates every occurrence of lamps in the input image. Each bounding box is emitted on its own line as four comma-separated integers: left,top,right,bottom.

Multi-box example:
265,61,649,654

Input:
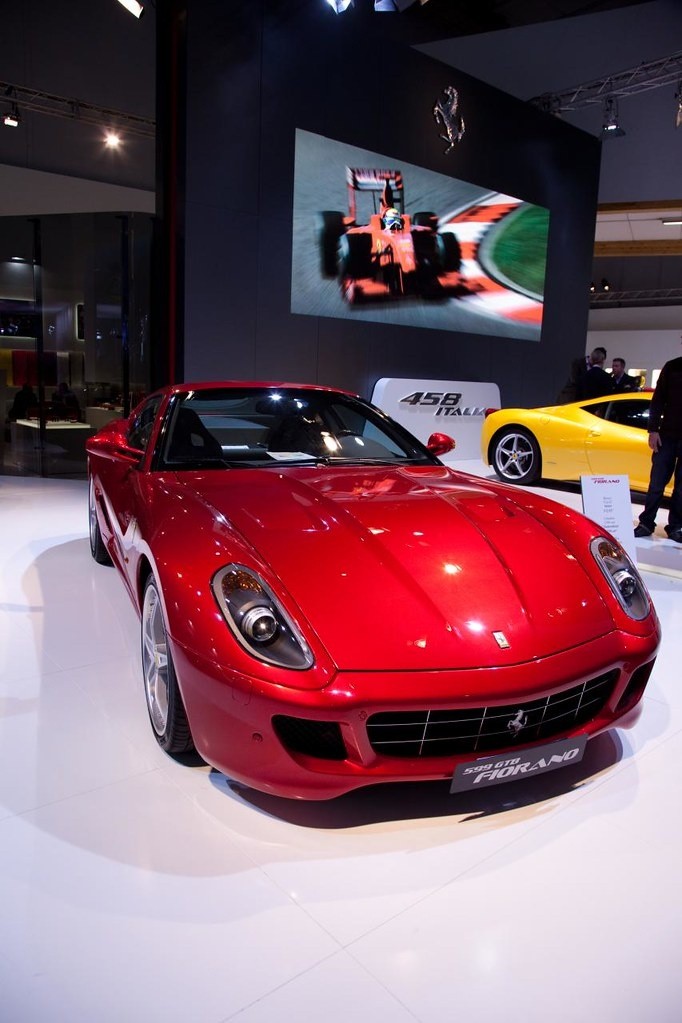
3,112,21,128
602,99,621,130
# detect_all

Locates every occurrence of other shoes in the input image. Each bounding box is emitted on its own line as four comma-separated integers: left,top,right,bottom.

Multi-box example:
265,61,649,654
668,530,682,543
634,526,652,537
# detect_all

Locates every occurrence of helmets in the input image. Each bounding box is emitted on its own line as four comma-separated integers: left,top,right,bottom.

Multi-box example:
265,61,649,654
383,208,403,230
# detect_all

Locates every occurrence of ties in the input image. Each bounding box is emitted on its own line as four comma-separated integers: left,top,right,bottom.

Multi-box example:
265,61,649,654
616,377,620,385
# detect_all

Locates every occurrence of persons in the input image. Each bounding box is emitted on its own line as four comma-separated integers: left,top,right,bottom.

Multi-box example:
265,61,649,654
570,347,617,415
602,358,635,420
634,357,682,542
7,383,39,423
59,383,81,421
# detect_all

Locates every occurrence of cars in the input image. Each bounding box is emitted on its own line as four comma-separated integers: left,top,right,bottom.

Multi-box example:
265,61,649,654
480,390,674,498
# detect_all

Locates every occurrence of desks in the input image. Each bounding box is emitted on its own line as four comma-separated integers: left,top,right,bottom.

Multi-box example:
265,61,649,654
17,418,96,475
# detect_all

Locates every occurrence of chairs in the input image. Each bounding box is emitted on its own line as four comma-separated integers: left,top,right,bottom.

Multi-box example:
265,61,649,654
142,402,226,471
267,407,340,460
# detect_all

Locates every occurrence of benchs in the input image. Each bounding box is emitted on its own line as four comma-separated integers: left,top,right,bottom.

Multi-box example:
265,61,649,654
5,399,53,418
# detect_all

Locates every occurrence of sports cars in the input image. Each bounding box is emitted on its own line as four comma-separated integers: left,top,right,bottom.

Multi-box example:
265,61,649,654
86,380,662,801
317,166,485,310
